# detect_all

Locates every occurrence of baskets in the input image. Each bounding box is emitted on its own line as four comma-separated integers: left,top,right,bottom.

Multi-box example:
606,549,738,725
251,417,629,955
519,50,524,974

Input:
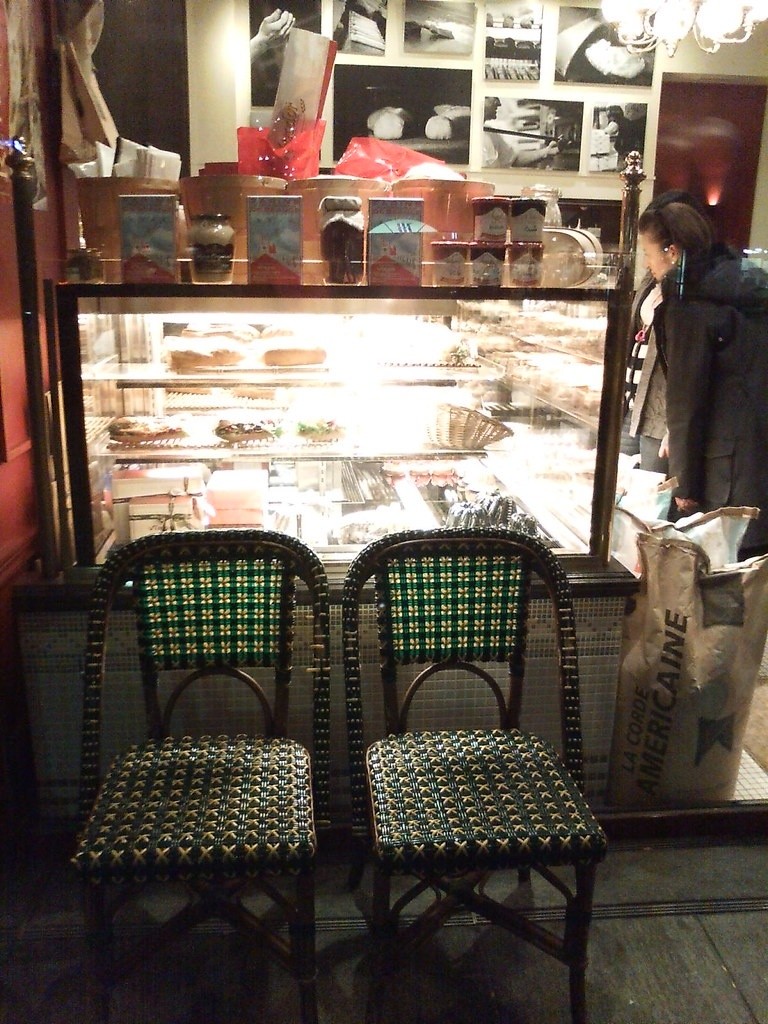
421,402,515,451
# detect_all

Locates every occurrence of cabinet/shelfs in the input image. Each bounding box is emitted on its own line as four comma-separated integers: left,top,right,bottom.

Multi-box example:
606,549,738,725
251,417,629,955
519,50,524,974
53,283,632,573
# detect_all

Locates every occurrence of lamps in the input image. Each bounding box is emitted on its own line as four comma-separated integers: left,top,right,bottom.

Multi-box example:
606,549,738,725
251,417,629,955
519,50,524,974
600,1,768,58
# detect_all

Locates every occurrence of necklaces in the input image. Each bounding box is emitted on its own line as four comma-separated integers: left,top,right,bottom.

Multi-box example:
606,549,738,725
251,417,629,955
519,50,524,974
634,287,653,342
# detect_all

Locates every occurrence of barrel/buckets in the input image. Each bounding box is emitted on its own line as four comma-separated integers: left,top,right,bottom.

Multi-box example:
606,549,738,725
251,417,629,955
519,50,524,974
179,173,288,282
392,179,496,285
288,179,393,284
78,176,179,283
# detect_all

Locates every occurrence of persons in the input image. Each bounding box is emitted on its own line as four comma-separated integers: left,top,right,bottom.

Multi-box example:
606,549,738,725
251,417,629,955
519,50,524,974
482,96,564,168
619,188,768,562
604,105,641,172
251,8,296,65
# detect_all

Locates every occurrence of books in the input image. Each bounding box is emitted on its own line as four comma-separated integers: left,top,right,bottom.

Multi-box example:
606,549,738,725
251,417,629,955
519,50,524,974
67,136,182,181
118,194,180,284
368,197,425,286
246,195,304,285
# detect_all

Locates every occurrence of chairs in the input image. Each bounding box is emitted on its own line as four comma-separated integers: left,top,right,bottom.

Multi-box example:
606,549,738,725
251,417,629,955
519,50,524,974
342,528,609,1024
61,530,332,1024
